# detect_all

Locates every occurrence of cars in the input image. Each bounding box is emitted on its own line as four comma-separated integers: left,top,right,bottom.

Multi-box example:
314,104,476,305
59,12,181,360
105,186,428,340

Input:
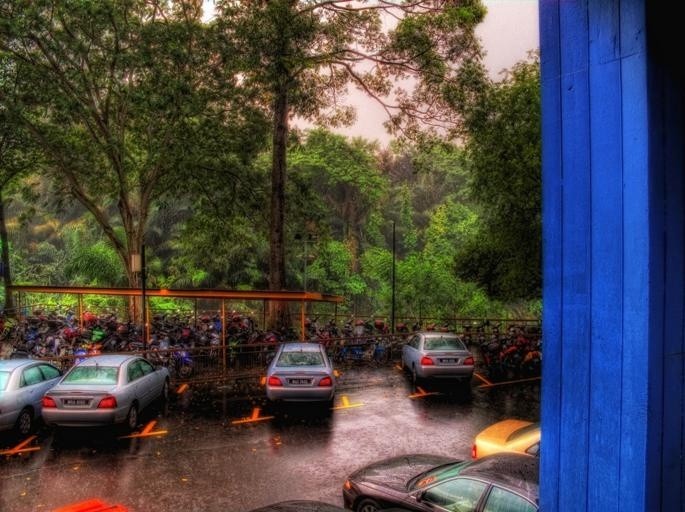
1,348,173,436
250,418,540,512
262,338,338,408
402,329,476,385
